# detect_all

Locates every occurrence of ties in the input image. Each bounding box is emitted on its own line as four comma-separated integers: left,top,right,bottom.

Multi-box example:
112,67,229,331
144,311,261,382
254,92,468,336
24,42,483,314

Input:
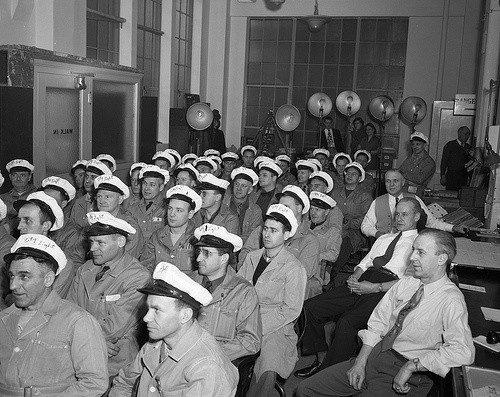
327,129,332,140
381,284,424,353
370,231,401,272
95,265,109,280
395,198,400,205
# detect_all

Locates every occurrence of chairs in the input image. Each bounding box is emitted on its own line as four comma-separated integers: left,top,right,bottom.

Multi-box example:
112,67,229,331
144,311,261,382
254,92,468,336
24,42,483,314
416,210,427,232
229,237,353,397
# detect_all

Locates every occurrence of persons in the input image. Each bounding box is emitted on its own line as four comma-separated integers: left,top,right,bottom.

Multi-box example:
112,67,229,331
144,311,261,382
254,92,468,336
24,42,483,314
317,118,344,153
350,118,380,169
397,131,435,197
440,126,474,190
0,145,475,397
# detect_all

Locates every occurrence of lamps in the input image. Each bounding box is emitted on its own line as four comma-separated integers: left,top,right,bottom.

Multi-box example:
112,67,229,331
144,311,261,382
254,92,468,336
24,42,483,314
274,90,428,158
482,134,500,165
186,102,214,156
269,0,285,5
301,0,329,33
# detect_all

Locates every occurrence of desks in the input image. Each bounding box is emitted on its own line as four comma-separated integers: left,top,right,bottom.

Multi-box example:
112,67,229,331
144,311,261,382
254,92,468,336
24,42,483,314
422,195,459,214
449,230,500,397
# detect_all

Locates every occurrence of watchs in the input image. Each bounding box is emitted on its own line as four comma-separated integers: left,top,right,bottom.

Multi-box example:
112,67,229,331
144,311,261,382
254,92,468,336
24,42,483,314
379,282,383,293
413,358,419,372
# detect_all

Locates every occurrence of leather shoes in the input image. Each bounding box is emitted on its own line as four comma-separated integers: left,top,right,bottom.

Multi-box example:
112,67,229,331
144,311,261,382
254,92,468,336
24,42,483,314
294,360,322,378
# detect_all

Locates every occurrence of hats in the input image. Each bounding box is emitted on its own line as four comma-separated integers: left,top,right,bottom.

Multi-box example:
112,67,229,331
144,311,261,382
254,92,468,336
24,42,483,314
4,144,372,236
409,132,428,143
3,233,68,276
136,261,213,311
194,222,243,253
